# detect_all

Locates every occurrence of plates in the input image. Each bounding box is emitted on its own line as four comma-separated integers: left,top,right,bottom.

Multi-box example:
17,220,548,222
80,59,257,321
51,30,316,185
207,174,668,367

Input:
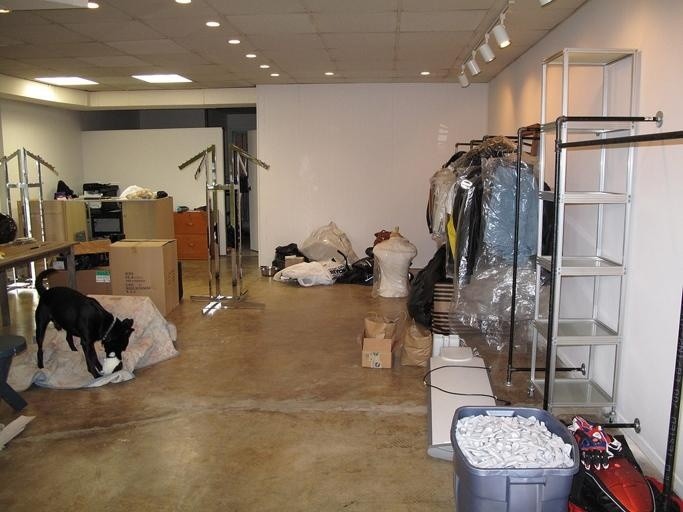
528,46,642,419
171,210,211,260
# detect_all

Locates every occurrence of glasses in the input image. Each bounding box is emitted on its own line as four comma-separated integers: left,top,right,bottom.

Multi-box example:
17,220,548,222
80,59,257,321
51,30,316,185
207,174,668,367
457,0,514,89
539,0,553,7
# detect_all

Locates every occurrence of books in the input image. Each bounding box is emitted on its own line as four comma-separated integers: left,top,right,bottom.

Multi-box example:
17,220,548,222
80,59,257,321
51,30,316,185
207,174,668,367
449,405,580,512
284,254,305,268
121,194,176,241
358,317,400,369
16,197,89,277
108,239,179,317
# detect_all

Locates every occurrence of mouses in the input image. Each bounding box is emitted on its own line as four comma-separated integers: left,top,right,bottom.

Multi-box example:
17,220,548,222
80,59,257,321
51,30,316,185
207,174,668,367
473,147,536,182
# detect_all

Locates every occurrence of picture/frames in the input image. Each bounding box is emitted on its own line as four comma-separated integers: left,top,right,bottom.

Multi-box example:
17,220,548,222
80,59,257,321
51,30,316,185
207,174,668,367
77,197,128,267
0,235,79,326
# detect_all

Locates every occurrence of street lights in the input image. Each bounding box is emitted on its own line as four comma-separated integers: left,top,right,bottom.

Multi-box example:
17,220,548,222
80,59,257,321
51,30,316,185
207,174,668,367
365,317,397,339
401,329,432,367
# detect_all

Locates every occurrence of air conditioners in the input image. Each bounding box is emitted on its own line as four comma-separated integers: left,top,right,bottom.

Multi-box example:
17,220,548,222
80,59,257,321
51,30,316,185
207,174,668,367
34,267,136,378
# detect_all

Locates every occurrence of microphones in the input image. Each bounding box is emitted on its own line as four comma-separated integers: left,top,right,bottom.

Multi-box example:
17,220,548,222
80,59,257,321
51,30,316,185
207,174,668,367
0,334,28,411
